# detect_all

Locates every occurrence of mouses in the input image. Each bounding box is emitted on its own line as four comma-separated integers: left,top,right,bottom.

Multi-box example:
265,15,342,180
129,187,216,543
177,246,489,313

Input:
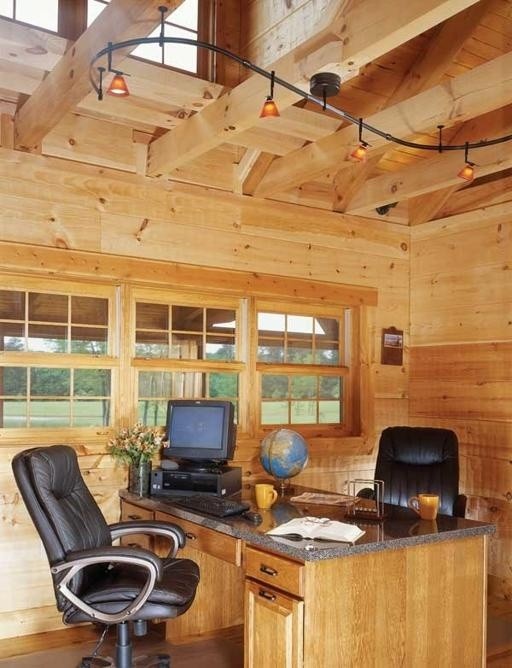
245,511,263,522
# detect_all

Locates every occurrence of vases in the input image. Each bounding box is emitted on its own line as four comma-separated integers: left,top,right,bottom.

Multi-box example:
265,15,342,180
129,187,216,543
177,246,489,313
129,461,152,495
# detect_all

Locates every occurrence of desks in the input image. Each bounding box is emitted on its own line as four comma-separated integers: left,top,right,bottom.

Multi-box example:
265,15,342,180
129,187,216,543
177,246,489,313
119,479,496,668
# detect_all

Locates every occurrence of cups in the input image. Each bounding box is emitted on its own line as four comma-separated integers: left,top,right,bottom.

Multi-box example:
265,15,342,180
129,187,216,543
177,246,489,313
408,494,439,519
255,483,277,509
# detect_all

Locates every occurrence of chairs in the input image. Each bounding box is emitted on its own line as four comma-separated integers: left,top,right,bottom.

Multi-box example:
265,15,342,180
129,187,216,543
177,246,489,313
12,445,200,668
356,427,467,518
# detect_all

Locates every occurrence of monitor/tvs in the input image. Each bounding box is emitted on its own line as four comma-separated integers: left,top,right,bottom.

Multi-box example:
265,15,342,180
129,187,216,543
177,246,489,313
161,400,236,460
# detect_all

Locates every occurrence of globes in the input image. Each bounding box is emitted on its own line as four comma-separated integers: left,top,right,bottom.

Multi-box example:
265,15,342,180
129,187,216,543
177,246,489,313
259,428,308,490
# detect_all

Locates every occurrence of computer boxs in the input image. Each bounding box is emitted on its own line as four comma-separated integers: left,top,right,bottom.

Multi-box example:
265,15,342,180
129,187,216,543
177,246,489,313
150,467,242,496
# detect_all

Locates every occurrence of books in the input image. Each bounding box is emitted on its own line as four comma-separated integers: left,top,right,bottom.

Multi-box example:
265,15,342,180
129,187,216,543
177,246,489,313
264,514,367,544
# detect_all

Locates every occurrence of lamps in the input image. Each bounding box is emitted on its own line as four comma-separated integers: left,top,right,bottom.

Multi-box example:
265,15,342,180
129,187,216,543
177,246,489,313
351,118,368,161
458,141,476,181
106,51,130,97
260,71,281,118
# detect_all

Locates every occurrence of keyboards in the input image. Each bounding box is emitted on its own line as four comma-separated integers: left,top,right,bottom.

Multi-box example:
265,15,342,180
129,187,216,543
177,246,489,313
177,494,249,518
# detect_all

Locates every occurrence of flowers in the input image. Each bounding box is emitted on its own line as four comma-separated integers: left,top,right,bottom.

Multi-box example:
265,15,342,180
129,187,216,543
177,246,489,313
108,423,166,464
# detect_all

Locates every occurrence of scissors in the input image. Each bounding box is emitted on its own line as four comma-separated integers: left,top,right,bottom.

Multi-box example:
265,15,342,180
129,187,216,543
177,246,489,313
266,533,303,541
